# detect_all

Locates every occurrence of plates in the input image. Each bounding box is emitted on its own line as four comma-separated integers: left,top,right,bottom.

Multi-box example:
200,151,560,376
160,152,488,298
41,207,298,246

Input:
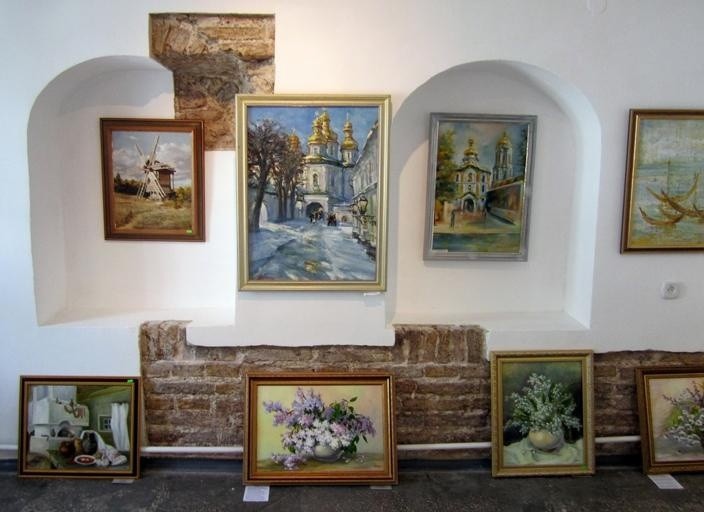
74,455,96,465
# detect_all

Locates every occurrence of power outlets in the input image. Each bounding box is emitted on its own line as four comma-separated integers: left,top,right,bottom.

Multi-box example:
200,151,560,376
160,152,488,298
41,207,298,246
660,281,680,300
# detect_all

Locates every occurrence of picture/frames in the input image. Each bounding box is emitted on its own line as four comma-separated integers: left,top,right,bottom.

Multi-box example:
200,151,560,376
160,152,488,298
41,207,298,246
235,92,392,293
634,365,704,474
242,369,398,487
99,116,205,242
422,111,537,261
18,374,142,478
620,109,704,253
488,350,595,477
